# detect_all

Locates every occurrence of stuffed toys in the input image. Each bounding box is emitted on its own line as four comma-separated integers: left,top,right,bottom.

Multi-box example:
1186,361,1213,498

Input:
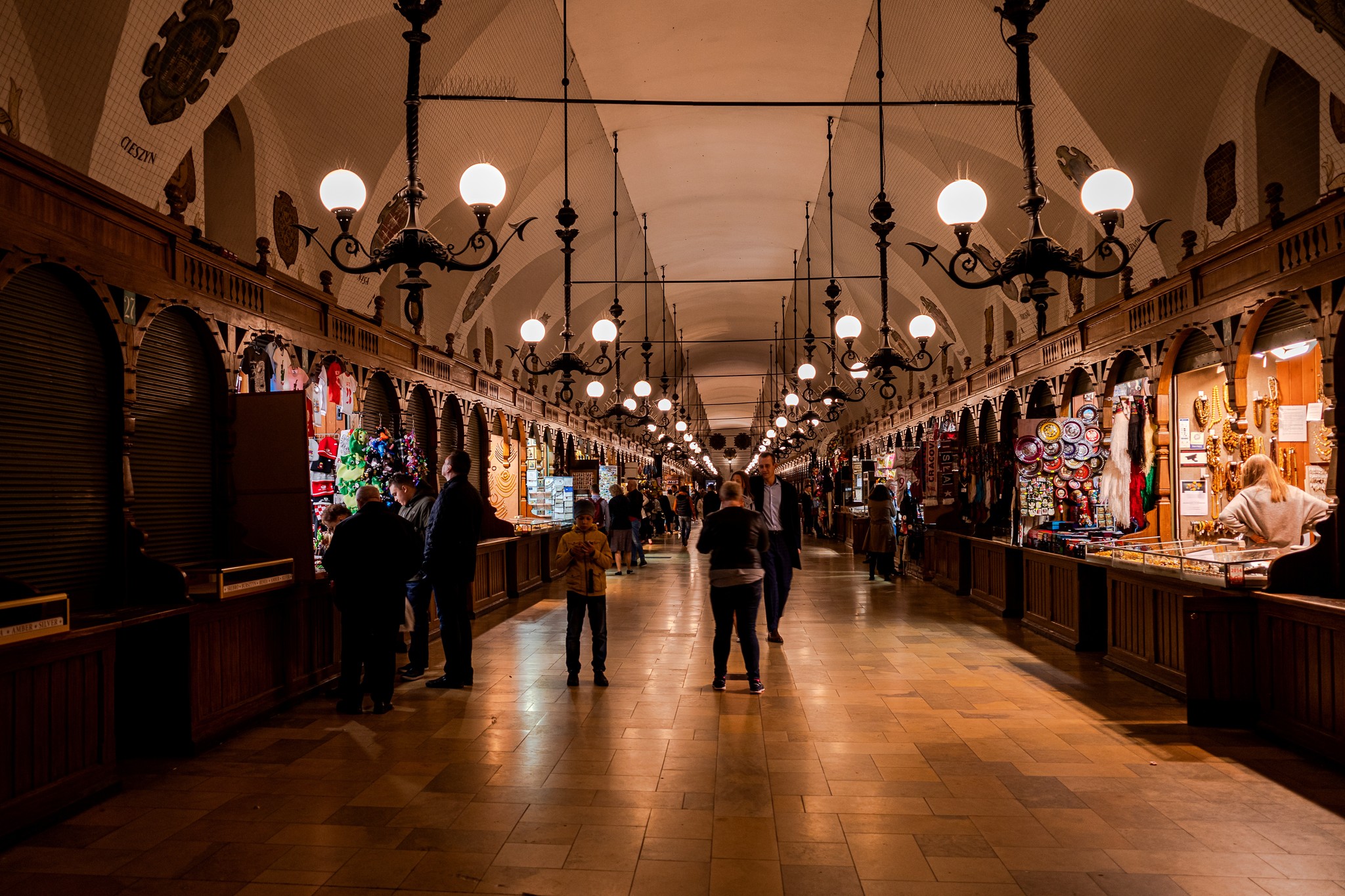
811,465,834,539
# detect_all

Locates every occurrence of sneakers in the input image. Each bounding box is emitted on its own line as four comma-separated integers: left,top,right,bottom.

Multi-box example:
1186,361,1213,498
712,676,726,690
397,663,429,672
400,668,424,680
750,679,765,694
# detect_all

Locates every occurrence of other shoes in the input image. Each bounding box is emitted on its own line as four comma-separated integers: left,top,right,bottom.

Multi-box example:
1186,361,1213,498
426,674,464,688
639,518,699,547
594,670,608,686
566,671,579,686
862,558,904,581
373,702,393,714
337,702,362,714
803,531,829,538
736,637,740,642
464,679,473,685
615,561,647,575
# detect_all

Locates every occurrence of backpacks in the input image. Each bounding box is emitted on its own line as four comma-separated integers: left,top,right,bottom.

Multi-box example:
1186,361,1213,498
588,497,605,529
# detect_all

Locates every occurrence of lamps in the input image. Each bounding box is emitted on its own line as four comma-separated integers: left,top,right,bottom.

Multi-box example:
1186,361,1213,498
290,0,1170,481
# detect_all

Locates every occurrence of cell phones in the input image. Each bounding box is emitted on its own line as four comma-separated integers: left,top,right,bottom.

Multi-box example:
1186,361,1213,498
581,540,593,550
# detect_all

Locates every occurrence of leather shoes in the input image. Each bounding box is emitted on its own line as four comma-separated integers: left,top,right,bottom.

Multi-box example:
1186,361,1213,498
767,631,784,642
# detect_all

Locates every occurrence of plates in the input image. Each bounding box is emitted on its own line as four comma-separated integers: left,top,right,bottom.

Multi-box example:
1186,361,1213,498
1015,404,1105,498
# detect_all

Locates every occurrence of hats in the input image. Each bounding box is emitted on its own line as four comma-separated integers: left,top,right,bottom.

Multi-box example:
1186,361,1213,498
573,499,595,519
308,436,337,532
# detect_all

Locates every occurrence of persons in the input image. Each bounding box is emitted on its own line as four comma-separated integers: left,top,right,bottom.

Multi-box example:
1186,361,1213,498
862,485,897,581
742,452,803,644
885,479,900,576
696,480,771,694
388,472,437,680
718,470,755,642
639,483,718,546
1218,453,1330,556
899,488,918,570
422,449,483,689
586,483,610,536
607,484,642,574
802,484,815,535
555,499,613,687
321,503,415,698
624,479,648,566
321,484,425,716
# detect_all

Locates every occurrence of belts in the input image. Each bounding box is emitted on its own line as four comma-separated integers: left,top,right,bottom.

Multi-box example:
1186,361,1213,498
768,530,783,534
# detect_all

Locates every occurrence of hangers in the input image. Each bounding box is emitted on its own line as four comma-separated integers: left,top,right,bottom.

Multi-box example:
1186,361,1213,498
271,330,276,350
279,335,284,352
252,333,262,356
